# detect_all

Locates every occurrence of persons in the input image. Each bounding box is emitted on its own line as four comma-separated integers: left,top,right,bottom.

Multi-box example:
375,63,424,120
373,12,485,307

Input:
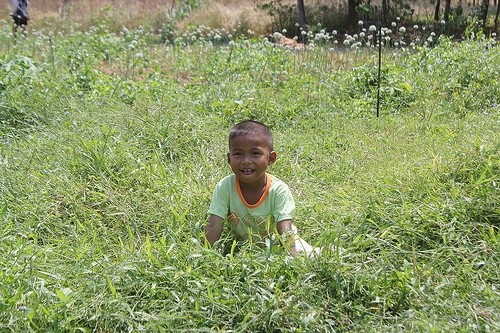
204,120,345,264
